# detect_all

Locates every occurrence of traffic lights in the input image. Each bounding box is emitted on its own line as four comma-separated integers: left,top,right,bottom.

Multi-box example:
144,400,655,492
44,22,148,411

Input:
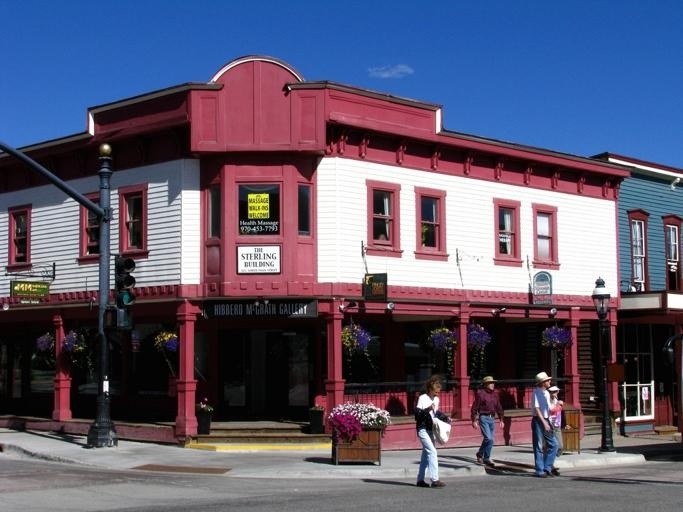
109,305,134,332
114,257,136,308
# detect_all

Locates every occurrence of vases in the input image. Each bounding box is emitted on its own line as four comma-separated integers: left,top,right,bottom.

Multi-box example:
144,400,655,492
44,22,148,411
306,405,324,433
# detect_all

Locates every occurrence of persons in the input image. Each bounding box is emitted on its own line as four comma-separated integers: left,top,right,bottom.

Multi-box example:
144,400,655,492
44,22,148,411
541,382,565,470
470,375,505,467
414,374,453,488
528,371,560,478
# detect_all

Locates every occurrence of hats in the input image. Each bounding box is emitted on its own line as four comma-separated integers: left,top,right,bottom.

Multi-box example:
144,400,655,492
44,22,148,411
481,376,498,382
548,385,560,391
535,372,552,386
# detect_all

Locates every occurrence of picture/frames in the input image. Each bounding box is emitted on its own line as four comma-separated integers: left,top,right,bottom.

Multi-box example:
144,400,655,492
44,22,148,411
194,409,212,435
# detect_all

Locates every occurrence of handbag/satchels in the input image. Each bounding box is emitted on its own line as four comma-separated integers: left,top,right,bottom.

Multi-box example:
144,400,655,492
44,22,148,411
432,417,451,444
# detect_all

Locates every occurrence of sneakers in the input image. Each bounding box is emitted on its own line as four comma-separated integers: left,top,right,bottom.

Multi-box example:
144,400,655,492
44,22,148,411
417,481,429,487
431,481,446,487
476,452,495,465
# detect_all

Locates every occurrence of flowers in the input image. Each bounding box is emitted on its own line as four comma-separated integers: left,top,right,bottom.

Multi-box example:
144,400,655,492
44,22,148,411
340,322,371,351
326,402,390,443
152,330,179,353
467,322,493,355
426,328,458,352
540,325,572,348
61,330,87,353
196,398,215,413
35,332,56,355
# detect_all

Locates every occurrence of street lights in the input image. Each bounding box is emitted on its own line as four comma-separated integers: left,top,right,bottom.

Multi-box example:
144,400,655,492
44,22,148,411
591,275,617,452
